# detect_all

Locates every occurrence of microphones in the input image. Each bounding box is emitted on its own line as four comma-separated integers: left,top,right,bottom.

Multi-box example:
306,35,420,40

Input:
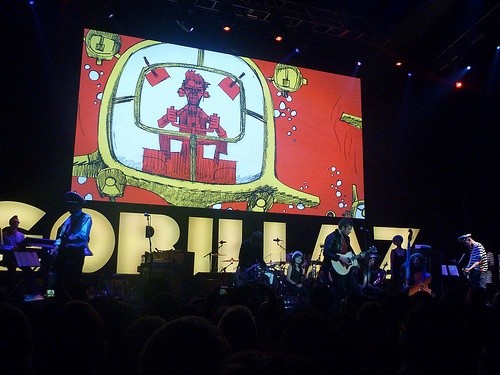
144,213,151,217
273,239,283,242
220,241,226,244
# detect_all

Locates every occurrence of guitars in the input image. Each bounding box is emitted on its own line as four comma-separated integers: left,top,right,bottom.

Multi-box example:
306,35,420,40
235,263,276,288
331,246,377,275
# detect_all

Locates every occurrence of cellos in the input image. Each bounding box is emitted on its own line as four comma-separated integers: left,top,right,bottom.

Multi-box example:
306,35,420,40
408,256,432,297
400,228,414,292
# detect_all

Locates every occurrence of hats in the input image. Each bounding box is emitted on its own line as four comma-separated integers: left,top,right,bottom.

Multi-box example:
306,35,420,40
410,253,424,260
458,234,471,244
63,192,85,205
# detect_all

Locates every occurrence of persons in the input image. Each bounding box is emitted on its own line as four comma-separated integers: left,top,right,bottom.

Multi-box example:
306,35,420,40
50,192,92,301
239,231,267,271
458,233,494,290
1,214,40,295
389,235,409,293
285,250,312,305
0,283,500,375
409,252,432,295
323,219,366,304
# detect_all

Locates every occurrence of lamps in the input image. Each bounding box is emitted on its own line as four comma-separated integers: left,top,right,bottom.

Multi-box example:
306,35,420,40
175,15,194,34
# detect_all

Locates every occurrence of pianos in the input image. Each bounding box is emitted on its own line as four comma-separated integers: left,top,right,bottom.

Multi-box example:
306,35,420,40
0,238,91,271
137,251,194,278
